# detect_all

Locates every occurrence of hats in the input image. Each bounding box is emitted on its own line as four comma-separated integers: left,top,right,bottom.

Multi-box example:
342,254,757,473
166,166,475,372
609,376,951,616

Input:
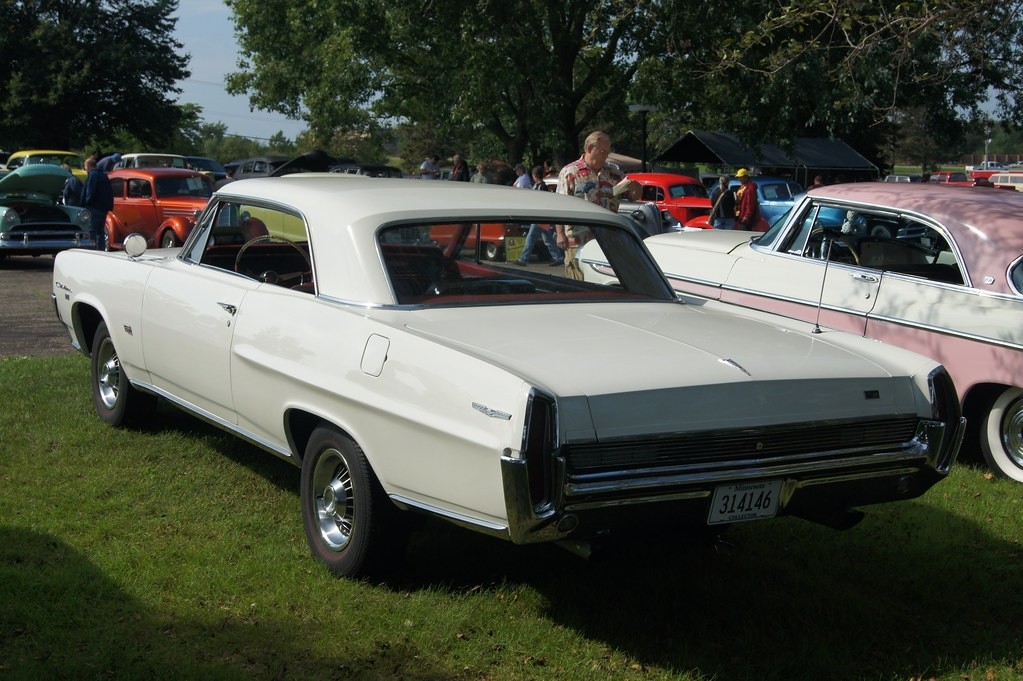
735,168,748,176
513,164,525,170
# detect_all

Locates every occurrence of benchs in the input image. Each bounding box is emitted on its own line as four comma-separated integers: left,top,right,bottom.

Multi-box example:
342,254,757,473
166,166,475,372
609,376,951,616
409,281,539,305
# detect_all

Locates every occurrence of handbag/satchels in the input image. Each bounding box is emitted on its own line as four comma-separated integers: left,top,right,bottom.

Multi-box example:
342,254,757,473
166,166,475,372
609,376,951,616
708,213,715,225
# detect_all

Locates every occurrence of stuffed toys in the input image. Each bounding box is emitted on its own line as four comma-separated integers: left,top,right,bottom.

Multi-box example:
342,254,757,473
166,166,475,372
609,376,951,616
841,211,858,233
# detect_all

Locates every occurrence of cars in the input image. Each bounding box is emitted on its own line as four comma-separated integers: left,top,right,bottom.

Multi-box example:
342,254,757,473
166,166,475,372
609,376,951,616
534,160,1023,490
0,150,505,267
53,173,970,578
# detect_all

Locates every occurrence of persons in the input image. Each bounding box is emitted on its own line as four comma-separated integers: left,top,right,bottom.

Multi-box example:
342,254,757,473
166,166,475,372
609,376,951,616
516,166,563,267
543,162,560,178
711,176,735,229
556,131,641,280
806,176,824,191
61,164,83,207
420,154,441,180
470,162,488,183
448,155,471,182
513,164,531,188
735,169,757,230
80,158,113,250
97,153,121,171
